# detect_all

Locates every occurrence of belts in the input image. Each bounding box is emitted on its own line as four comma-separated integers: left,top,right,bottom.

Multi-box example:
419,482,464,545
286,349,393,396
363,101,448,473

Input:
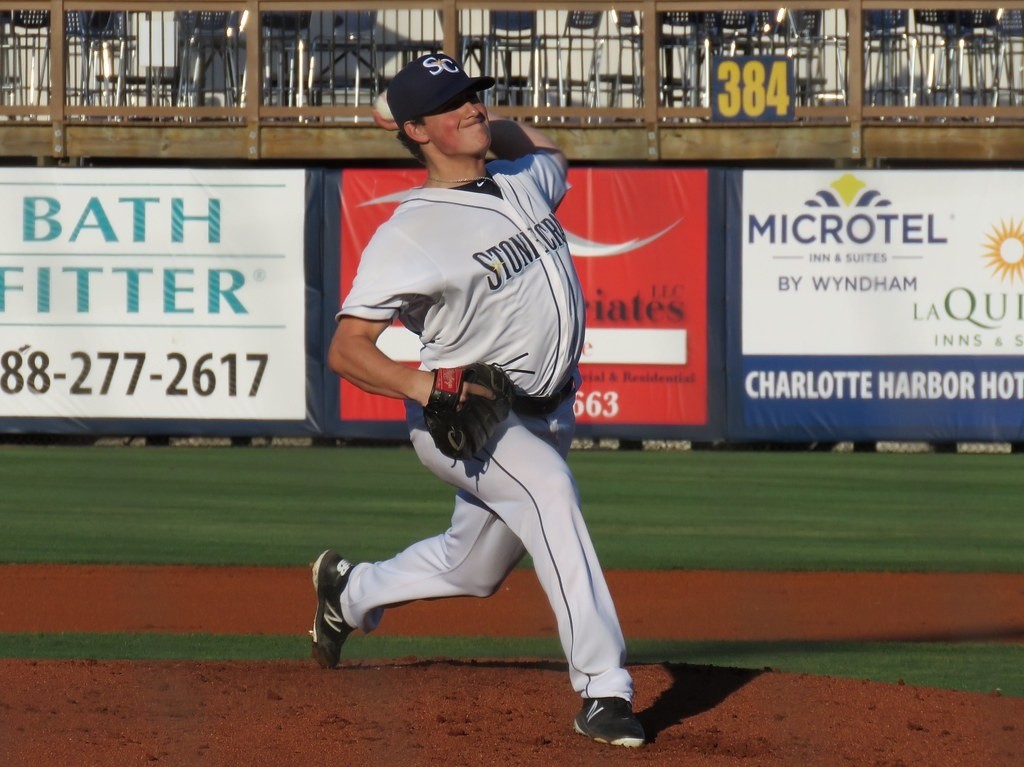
511,377,574,414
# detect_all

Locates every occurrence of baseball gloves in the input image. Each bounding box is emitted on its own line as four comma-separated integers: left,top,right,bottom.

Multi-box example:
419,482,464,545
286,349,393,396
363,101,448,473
422,361,516,461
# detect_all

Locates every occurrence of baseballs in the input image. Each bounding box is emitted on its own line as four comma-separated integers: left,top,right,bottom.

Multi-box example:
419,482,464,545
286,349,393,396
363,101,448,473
375,89,396,122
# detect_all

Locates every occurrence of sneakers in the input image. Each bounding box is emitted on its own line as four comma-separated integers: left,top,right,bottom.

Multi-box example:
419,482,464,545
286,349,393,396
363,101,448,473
574,697,646,748
308,549,357,670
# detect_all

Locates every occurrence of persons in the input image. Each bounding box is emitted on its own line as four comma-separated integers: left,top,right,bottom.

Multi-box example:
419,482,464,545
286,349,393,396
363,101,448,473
311,53,646,748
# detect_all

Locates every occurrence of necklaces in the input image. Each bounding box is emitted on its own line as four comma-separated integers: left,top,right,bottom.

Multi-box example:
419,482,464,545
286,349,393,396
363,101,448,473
428,175,497,185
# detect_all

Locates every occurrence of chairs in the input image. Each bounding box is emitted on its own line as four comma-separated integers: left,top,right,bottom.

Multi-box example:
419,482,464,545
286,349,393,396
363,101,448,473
0,9,1024,125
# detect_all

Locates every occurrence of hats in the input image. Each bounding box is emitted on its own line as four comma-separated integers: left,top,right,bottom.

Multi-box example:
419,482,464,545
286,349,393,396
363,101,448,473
387,53,496,128
